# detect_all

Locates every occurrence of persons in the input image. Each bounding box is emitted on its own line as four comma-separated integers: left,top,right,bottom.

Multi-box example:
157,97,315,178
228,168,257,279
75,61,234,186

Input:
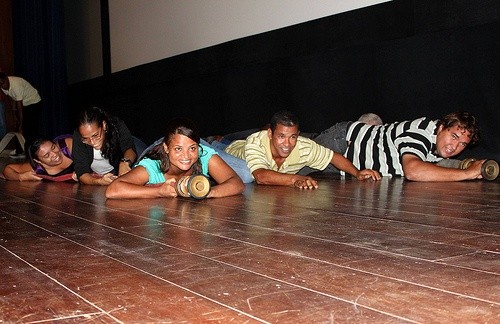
295,113,488,182
3,134,79,182
358,114,383,125
105,123,254,199
0,76,43,159
210,111,382,190
72,106,148,185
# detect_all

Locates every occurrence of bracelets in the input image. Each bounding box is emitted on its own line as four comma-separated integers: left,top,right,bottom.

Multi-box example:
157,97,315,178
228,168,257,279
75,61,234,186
121,157,133,166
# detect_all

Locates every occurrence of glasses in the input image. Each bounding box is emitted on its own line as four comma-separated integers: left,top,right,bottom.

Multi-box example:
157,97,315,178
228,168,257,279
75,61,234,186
81,126,103,143
